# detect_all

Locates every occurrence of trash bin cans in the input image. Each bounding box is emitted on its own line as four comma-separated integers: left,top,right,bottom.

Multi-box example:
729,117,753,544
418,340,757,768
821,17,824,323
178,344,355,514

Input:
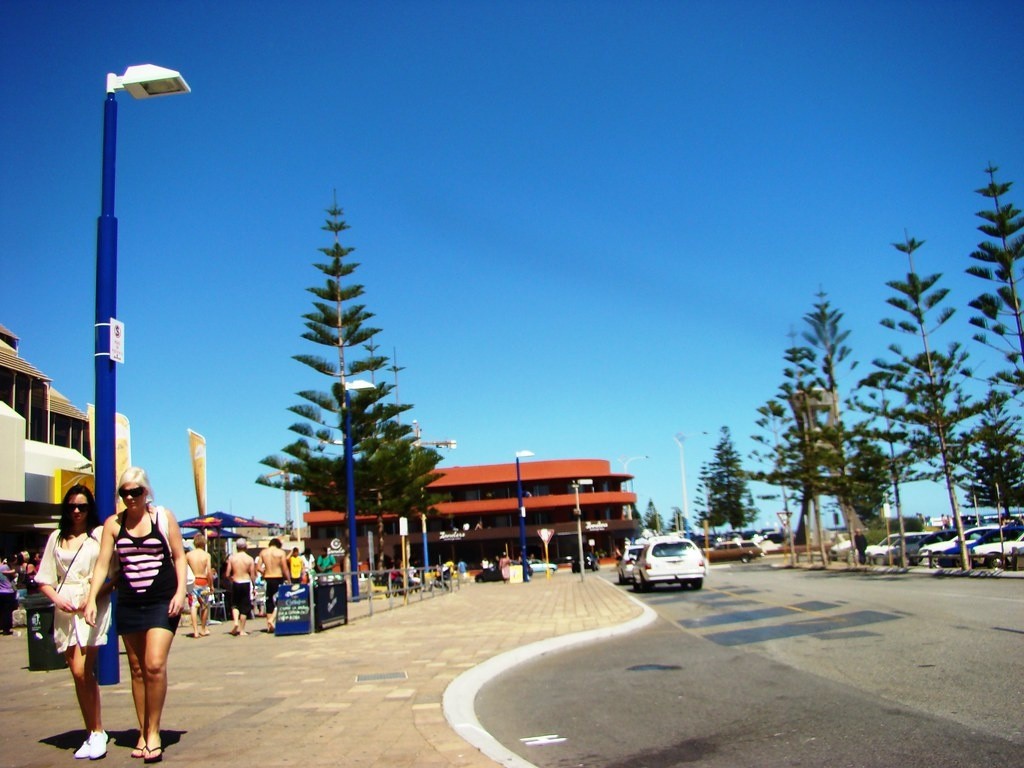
18,592,70,671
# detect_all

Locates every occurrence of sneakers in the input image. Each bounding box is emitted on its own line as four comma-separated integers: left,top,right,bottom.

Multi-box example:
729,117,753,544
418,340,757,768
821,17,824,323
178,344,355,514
74,729,109,759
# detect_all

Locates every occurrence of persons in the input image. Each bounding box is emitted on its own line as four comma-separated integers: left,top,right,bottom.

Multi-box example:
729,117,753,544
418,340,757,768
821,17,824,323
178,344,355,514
317,547,336,574
226,538,257,635
0,550,44,636
387,559,467,594
257,538,291,633
854,528,868,565
34,484,116,760
287,547,315,585
499,551,512,585
84,467,188,764
186,533,215,638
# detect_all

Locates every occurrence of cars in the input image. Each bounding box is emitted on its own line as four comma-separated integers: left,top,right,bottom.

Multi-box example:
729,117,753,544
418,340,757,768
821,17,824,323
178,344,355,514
865,531,932,566
632,538,705,592
917,526,999,567
616,547,638,585
883,530,959,567
972,533,1024,571
939,526,1024,567
694,527,851,564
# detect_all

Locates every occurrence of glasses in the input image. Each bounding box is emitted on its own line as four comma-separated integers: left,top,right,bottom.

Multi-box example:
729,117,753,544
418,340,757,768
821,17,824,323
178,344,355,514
69,502,89,512
119,486,145,497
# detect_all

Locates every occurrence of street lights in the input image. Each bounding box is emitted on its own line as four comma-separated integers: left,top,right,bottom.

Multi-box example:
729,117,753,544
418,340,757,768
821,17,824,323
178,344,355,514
572,479,593,584
94,63,193,688
514,449,534,582
344,379,376,601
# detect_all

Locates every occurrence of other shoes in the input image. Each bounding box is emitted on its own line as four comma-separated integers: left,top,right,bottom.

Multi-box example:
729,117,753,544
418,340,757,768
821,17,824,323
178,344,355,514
3,631,13,635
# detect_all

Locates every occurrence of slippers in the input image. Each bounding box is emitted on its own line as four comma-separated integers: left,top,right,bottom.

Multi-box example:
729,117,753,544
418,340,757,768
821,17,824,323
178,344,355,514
131,747,145,758
145,746,162,762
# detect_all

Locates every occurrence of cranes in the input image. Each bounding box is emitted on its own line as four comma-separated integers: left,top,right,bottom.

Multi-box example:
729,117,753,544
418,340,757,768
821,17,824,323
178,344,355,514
318,421,458,453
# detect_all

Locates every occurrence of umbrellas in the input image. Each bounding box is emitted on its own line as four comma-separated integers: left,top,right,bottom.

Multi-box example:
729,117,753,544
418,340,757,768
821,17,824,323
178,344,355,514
176,511,284,606
